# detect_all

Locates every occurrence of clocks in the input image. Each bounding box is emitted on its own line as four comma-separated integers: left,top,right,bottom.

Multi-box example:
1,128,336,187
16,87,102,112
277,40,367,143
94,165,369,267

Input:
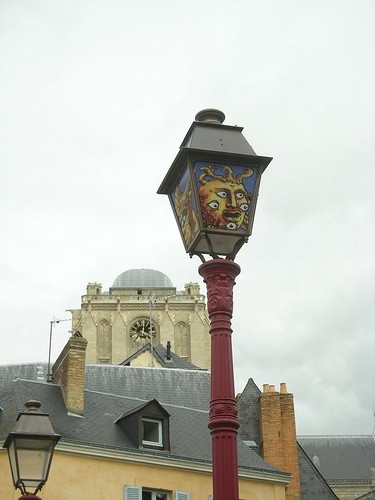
130,319,156,345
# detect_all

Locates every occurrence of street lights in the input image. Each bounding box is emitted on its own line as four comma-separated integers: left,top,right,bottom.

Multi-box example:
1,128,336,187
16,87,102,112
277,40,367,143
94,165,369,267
155,109,274,500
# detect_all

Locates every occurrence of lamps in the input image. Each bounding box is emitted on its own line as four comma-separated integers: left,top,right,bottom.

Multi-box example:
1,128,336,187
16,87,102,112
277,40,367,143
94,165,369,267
2,399,63,500
